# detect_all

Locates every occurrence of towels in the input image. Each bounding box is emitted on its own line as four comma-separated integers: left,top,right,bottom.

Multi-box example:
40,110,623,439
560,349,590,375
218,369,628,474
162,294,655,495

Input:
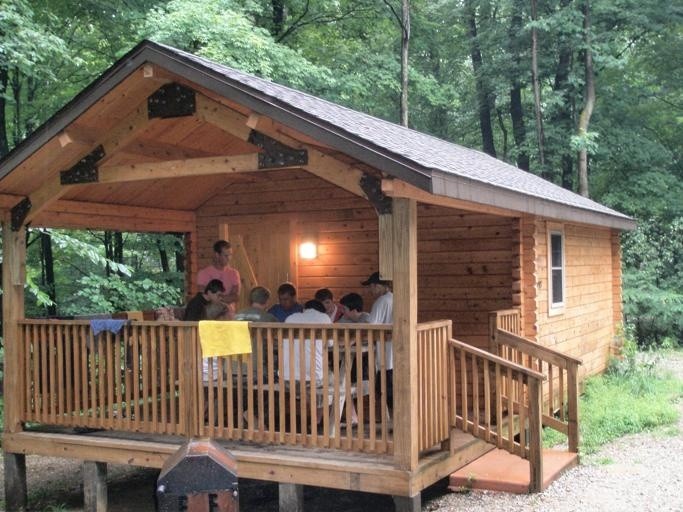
196,319,252,358
89,318,133,335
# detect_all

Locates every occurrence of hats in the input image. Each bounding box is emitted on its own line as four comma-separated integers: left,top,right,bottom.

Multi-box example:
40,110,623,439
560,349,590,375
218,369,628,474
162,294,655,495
360,271,389,286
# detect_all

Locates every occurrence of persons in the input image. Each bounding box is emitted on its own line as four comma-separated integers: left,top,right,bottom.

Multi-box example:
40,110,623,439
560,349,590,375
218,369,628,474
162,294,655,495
221,286,282,431
190,300,228,422
359,270,393,434
184,278,223,323
194,241,240,314
266,283,303,322
314,287,343,322
330,292,372,429
277,300,334,431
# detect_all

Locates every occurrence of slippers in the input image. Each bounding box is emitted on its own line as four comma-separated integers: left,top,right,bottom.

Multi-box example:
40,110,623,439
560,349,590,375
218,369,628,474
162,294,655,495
243,415,392,434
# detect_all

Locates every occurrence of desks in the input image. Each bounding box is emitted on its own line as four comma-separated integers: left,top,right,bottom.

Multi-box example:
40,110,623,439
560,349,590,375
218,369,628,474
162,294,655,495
258,341,376,439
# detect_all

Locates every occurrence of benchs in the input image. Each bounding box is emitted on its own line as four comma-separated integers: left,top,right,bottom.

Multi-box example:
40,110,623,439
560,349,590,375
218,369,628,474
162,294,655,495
174,377,357,437
